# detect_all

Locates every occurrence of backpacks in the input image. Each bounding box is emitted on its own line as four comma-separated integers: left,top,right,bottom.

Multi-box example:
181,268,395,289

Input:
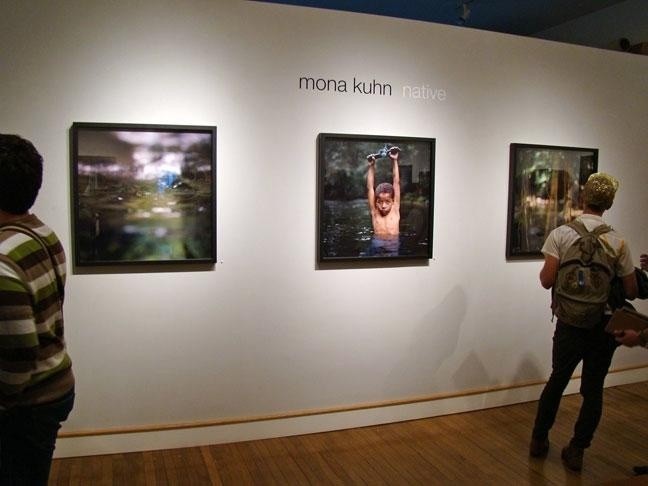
551,222,617,331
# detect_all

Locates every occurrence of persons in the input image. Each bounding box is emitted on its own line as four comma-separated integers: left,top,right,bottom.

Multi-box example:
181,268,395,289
0,132,76,486
613,253,648,349
365,147,402,257
527,171,637,471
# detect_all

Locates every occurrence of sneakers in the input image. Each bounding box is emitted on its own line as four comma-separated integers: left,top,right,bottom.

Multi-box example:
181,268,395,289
529,437,550,458
562,445,584,473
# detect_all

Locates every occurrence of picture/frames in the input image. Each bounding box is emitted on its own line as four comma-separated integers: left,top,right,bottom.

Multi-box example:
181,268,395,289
68,120,216,269
503,141,599,260
315,131,436,262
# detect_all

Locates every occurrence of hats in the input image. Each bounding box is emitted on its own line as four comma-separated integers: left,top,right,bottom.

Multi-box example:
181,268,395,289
581,173,618,206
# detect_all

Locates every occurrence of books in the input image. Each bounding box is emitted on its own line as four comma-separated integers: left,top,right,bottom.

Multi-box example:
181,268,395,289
604,307,647,334
